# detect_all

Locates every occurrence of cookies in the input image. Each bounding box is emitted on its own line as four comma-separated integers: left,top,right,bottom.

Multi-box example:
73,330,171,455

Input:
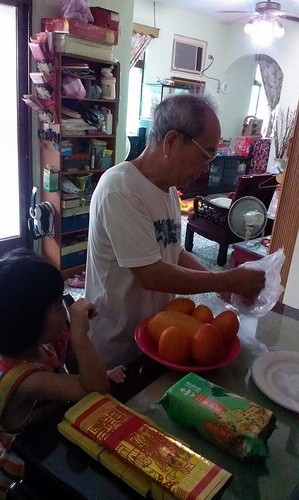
217,402,271,438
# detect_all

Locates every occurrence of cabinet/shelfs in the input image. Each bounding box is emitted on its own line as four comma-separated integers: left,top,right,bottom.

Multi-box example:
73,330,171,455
37,51,121,273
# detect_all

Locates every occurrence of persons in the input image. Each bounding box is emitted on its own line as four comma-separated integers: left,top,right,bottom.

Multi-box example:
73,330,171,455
83,93,267,372
0,247,111,499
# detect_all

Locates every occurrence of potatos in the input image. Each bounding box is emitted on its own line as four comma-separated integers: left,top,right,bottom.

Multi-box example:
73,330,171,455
147,297,240,365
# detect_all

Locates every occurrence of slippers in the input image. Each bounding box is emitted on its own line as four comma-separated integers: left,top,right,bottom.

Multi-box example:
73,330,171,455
66,271,86,288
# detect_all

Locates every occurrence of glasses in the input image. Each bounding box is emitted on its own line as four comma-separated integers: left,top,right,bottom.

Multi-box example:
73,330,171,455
192,138,220,164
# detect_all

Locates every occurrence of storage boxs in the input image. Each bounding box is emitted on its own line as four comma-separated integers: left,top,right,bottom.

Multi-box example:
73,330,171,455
41,6,120,45
62,194,89,269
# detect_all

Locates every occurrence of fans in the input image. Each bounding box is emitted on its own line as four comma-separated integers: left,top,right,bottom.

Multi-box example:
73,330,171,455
219,0,299,24
228,196,268,247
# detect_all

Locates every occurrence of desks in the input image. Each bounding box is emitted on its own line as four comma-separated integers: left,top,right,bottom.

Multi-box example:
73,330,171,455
189,155,241,194
10,301,299,500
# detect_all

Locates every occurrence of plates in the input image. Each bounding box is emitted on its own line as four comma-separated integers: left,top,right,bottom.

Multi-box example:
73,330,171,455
251,351,299,413
134,312,243,371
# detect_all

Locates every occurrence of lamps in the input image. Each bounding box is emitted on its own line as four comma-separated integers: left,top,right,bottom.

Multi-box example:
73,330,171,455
244,18,285,47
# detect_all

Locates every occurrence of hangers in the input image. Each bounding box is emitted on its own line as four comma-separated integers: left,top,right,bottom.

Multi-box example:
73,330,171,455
258,172,286,188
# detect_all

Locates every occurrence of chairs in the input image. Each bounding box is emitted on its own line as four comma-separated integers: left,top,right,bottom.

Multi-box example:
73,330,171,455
185,173,278,267
